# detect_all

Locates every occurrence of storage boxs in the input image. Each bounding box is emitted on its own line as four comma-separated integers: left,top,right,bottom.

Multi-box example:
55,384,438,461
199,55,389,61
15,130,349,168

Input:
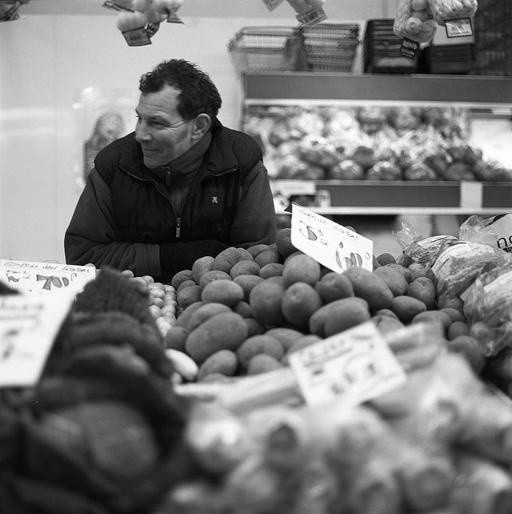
227,15,476,74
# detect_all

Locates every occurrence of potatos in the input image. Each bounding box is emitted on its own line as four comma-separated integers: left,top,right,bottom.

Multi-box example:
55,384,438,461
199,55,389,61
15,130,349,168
164,227,487,381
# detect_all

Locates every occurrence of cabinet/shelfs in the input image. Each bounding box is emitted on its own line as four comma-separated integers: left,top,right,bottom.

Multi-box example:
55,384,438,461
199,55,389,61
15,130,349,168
236,67,512,258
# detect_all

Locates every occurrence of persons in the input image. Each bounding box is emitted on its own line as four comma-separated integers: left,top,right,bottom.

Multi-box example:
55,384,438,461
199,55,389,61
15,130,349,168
64,59,278,284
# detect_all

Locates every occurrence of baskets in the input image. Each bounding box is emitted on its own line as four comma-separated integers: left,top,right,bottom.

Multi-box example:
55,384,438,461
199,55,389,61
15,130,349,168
227,19,476,73
